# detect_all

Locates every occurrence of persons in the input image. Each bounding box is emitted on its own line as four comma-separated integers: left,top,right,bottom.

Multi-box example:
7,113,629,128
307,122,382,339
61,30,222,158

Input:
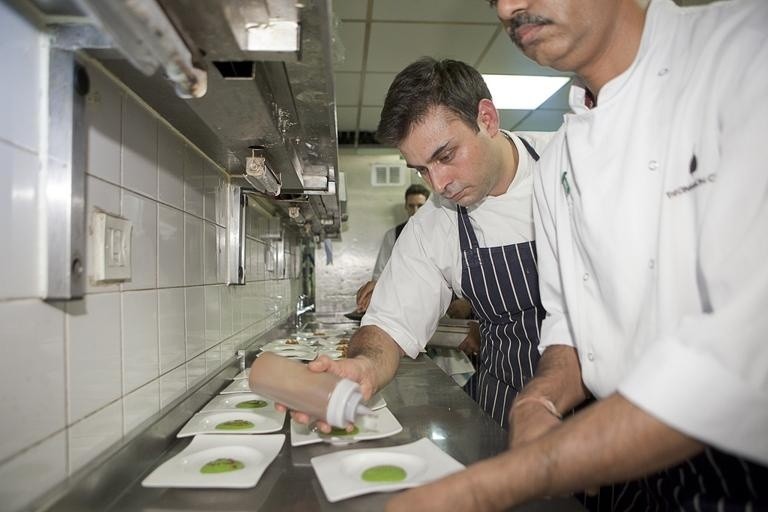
387,0,766,511
353,182,480,402
272,56,591,434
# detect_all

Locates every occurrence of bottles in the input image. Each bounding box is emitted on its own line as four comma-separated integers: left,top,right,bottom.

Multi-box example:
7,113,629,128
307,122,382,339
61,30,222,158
249,351,373,428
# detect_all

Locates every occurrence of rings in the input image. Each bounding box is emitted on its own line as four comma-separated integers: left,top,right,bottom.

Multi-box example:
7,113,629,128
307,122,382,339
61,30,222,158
473,351,479,356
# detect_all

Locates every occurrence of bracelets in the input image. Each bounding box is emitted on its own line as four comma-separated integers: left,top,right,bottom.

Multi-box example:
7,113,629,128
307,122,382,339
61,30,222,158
506,394,562,422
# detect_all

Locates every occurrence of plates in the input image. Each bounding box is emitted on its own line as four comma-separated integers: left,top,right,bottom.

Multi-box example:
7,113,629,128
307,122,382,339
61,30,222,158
371,397,390,411
220,379,253,393
312,437,467,503
176,411,286,439
255,326,350,364
289,406,402,446
141,434,286,490
232,368,253,379
199,394,277,410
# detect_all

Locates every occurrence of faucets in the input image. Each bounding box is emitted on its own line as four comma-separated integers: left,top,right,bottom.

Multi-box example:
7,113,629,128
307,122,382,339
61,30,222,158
295,293,315,323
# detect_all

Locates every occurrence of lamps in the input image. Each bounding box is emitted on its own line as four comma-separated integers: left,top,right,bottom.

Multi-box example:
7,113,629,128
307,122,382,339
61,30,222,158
72,0,207,100
242,146,322,249
